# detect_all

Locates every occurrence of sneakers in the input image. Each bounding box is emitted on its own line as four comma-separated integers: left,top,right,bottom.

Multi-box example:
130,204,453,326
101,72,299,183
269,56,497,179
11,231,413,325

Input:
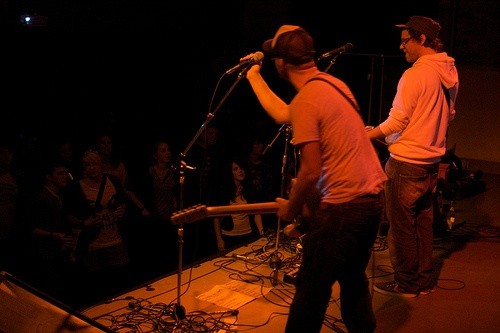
373,280,417,298
420,288,430,295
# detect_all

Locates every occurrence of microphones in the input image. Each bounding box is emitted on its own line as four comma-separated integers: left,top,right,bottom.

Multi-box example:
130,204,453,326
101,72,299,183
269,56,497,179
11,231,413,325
226,51,264,75
320,43,353,59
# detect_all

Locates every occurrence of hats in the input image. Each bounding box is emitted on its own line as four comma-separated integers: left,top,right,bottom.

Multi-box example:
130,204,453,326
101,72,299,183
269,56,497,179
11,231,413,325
262,24,316,62
394,16,441,34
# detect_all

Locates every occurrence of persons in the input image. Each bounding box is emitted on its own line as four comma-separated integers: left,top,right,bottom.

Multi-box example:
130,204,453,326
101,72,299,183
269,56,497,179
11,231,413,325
97,122,221,225
28,167,74,251
365,15,460,298
240,25,390,333
60,151,126,252
210,146,266,253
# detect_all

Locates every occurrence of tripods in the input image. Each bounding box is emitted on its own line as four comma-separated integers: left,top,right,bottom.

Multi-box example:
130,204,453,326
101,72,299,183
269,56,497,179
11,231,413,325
129,69,238,333
232,53,348,286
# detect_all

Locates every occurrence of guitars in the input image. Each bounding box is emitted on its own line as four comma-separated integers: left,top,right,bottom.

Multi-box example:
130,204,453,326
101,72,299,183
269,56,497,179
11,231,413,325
170,189,386,232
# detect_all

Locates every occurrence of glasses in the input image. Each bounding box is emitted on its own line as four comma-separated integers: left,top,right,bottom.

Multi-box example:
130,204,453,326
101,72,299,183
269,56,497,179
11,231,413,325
400,35,415,44
